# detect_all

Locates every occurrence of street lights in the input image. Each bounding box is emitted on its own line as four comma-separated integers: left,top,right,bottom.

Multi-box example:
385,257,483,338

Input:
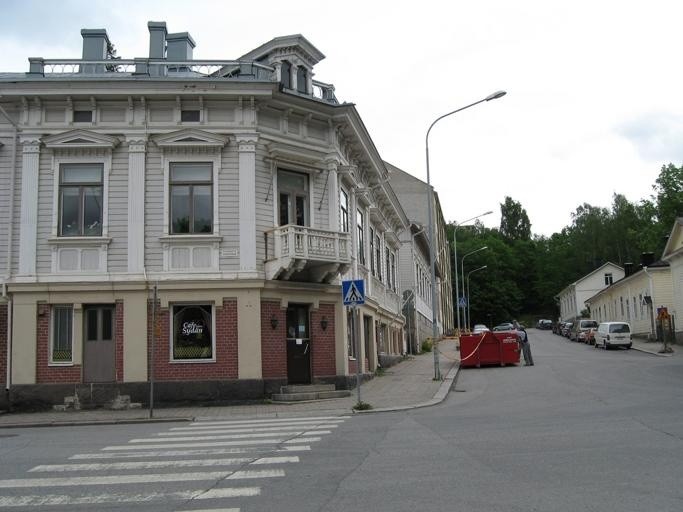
467,266,487,333
454,211,493,337
426,90,508,380
462,246,488,333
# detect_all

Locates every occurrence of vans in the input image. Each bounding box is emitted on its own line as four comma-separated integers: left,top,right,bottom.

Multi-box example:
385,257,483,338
536,319,639,350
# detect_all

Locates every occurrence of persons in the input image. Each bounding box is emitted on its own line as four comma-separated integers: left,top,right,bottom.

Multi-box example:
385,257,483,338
514,320,534,366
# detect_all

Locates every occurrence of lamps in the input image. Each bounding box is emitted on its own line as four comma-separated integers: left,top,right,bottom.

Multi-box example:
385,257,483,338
319,315,328,331
270,314,279,329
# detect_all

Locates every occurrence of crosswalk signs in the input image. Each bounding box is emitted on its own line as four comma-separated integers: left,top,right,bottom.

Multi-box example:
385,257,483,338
341,279,365,305
458,297,467,307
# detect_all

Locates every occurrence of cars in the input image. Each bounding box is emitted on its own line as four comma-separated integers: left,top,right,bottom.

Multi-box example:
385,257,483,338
492,322,514,331
473,325,490,334
